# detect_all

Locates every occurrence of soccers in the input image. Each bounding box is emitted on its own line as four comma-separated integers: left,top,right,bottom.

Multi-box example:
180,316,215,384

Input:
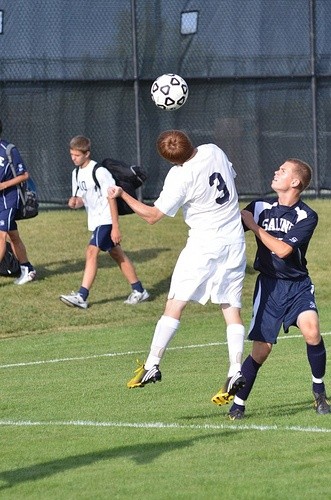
150,73,189,111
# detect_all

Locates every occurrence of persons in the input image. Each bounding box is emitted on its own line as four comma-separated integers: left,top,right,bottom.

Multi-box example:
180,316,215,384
229,158,331,419
59,136,149,309
0,139,37,286
107,129,247,406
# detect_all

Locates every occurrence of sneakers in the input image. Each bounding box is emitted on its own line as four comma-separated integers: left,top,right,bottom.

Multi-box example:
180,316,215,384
313,391,331,415
127,359,161,389
124,289,149,304
227,409,244,420
15,266,37,285
211,371,246,407
59,291,88,309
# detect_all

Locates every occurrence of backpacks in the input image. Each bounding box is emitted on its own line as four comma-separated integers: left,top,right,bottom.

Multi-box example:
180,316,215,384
0,242,21,276
76,158,147,215
6,144,39,220
